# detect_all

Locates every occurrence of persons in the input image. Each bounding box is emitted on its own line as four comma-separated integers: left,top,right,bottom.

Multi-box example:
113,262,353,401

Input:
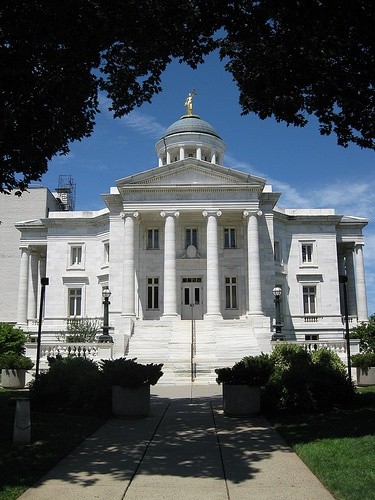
185,93,192,115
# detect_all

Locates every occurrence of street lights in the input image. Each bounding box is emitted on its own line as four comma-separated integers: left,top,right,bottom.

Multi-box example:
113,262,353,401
35,277,50,376
339,274,352,381
99,289,114,343
271,287,286,341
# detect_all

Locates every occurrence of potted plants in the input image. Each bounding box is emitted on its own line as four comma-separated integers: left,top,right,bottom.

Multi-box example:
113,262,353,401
214,362,261,416
0,352,34,388
351,353,375,385
99,358,164,419
